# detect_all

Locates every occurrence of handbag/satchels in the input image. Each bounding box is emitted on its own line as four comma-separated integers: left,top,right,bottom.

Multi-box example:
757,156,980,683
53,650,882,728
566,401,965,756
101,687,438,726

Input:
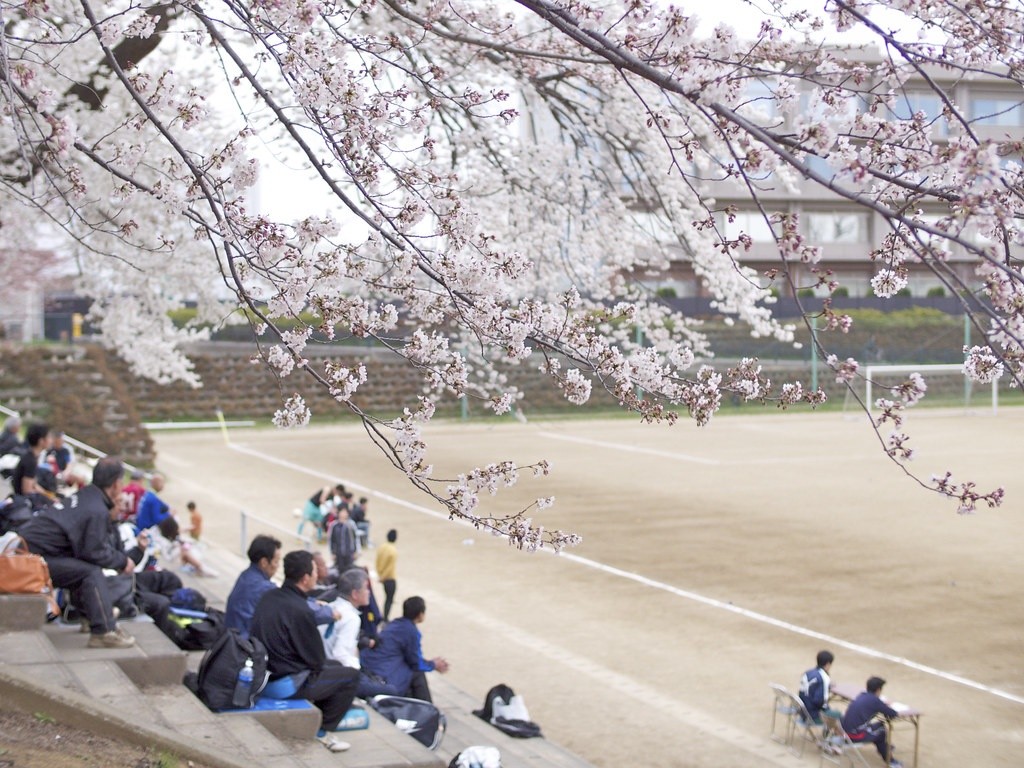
0,537,61,615
480,684,542,738
170,588,206,611
334,704,368,731
367,695,446,751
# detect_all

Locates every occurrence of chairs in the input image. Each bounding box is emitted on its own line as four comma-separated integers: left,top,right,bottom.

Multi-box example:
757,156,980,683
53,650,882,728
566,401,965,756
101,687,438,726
767,682,841,759
819,710,876,768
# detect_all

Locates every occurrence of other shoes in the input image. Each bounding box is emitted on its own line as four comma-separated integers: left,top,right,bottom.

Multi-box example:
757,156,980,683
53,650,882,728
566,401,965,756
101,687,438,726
890,746,894,750
197,569,218,577
824,745,842,755
888,760,903,768
177,535,195,544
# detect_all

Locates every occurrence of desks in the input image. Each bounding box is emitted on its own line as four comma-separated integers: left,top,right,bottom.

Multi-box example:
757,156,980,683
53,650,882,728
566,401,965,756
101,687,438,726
830,686,926,768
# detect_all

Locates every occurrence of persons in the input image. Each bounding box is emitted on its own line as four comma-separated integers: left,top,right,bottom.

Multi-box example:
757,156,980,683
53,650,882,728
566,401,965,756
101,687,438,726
842,676,908,768
0,416,219,648
73,312,83,338
797,651,845,755
226,482,449,750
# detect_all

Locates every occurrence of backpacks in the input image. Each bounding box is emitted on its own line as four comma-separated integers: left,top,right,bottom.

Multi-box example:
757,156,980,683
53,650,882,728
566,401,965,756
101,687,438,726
198,628,272,712
155,606,225,649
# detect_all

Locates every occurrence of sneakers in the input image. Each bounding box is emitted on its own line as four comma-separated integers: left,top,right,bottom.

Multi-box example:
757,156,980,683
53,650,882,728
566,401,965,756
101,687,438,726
80,607,120,633
315,733,349,751
88,629,136,648
